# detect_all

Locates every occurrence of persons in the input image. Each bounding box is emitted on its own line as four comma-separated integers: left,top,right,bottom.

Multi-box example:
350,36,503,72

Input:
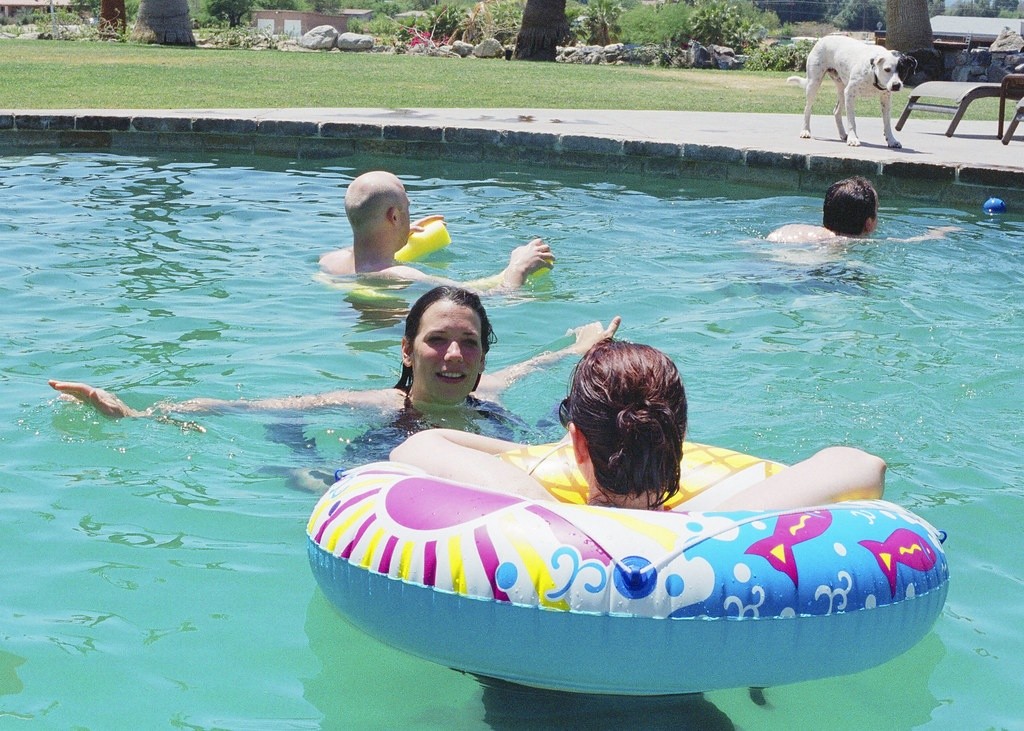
388,338,887,511
319,171,556,286
765,176,880,244
48,286,621,417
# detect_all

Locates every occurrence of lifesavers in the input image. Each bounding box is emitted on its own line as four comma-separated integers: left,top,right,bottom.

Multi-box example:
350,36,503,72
309,218,556,305
303,439,951,698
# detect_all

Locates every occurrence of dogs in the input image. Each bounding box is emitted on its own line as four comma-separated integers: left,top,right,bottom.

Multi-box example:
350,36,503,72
786,34,918,149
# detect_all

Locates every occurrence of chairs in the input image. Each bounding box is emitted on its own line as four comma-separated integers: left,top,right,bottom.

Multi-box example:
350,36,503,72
1001,97,1024,145
895,62,1024,139
